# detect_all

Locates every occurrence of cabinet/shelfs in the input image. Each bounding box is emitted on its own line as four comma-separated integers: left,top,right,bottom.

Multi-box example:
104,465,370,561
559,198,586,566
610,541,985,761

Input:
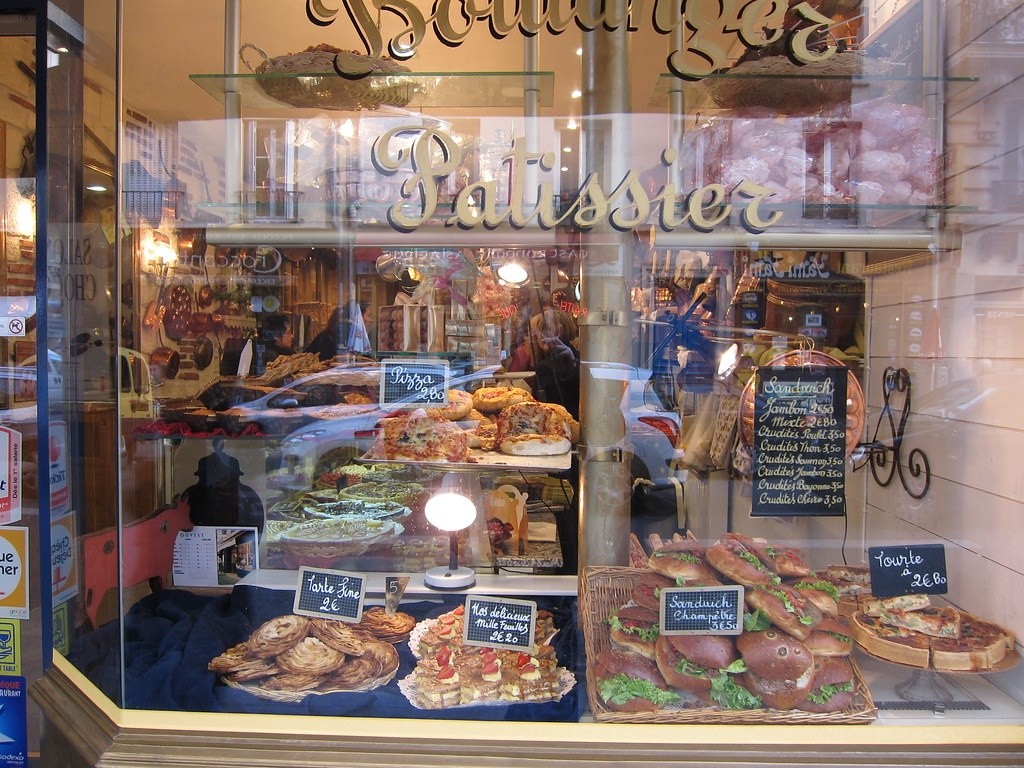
184,69,556,219
652,67,981,216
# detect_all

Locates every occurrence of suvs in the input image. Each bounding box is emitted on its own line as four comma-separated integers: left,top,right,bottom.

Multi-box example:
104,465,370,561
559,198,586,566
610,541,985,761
275,361,688,521
233,361,467,443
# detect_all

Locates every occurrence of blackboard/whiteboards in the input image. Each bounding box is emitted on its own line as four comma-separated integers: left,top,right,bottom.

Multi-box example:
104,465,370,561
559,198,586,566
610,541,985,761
292,565,367,623
377,357,450,408
659,586,744,636
867,542,949,599
462,594,537,653
750,363,851,517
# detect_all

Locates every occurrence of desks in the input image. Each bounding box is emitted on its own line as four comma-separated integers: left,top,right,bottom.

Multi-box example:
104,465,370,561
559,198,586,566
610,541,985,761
355,512,562,575
136,431,396,502
362,435,579,577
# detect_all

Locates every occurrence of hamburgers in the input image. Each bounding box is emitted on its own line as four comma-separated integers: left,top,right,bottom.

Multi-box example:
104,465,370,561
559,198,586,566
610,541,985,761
594,531,854,713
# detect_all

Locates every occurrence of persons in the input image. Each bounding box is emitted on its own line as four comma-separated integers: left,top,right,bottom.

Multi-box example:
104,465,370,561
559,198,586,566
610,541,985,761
252,314,298,387
506,285,580,604
300,299,371,365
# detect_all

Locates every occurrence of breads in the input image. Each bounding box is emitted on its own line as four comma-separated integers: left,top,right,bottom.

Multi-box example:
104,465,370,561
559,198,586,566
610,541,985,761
371,386,580,464
206,607,415,691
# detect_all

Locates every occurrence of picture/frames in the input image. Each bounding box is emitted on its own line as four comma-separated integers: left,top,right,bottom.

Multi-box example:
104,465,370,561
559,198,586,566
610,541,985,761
852,0,944,277
732,251,842,361
800,120,862,221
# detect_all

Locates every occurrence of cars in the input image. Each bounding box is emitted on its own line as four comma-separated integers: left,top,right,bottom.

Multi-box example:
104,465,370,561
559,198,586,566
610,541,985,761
846,379,993,475
119,348,154,430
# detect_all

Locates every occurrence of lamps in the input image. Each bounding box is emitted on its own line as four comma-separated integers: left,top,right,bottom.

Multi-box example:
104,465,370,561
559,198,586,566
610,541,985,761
415,474,478,591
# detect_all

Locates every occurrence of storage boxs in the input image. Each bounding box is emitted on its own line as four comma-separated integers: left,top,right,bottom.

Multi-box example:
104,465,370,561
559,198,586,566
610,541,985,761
447,338,489,355
377,305,421,352
421,304,445,352
445,319,486,337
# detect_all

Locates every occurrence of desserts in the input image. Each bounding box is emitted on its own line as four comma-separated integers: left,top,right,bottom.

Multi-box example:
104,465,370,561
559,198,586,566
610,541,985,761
263,463,443,555
416,606,561,708
817,566,1015,670
